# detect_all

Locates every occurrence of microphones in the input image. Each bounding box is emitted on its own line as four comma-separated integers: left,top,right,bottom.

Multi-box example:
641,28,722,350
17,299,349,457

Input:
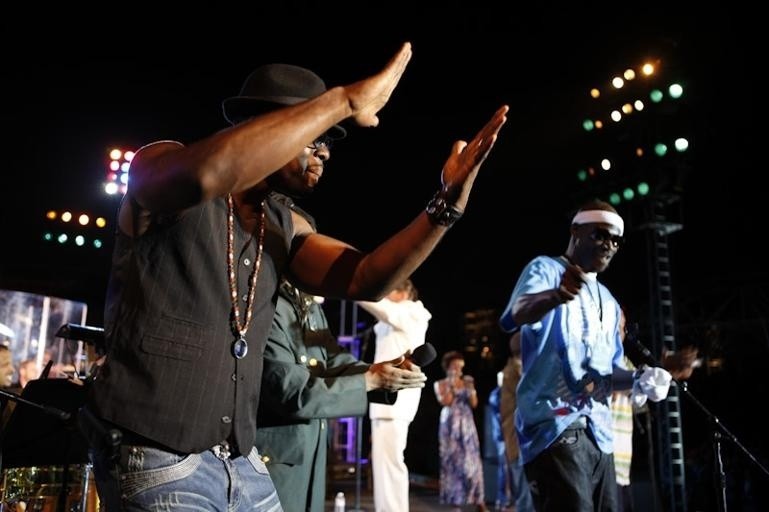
396,343,436,371
625,326,652,357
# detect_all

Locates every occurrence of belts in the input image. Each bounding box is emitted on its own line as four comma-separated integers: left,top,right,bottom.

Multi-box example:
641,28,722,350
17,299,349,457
561,415,591,433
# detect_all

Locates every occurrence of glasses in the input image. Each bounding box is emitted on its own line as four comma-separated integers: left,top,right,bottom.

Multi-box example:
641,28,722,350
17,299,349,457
591,228,626,250
312,133,335,149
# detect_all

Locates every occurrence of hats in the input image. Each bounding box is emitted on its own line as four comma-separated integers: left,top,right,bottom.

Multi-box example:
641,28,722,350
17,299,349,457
220,62,354,141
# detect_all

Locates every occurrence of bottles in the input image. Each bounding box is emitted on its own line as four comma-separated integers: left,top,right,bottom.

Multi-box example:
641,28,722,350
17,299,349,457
79,462,100,512
335,493,345,512
